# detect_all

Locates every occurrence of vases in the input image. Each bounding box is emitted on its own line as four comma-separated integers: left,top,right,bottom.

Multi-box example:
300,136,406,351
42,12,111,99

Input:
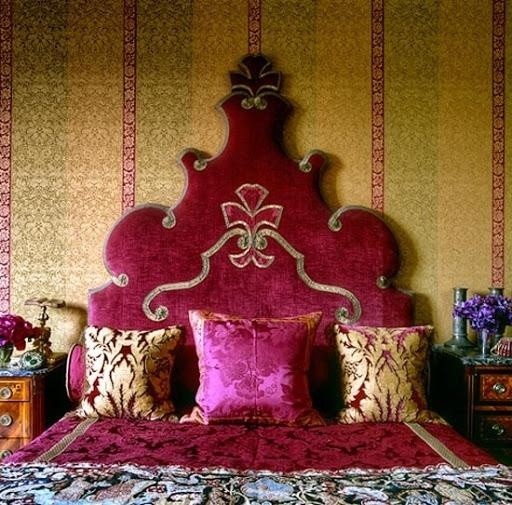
444,285,505,360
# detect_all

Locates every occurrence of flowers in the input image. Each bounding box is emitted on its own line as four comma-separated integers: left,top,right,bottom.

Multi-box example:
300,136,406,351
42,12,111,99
1,315,42,364
448,294,512,331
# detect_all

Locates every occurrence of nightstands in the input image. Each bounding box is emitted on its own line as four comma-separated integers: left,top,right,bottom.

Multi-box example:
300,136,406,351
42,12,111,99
429,344,512,472
0,351,68,466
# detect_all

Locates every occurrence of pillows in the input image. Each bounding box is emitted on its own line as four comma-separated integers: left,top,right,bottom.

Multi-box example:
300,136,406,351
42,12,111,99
331,322,439,425
74,325,186,425
179,308,328,428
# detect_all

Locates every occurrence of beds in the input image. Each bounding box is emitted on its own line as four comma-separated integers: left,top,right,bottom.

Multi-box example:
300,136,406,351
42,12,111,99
0,48,512,504
